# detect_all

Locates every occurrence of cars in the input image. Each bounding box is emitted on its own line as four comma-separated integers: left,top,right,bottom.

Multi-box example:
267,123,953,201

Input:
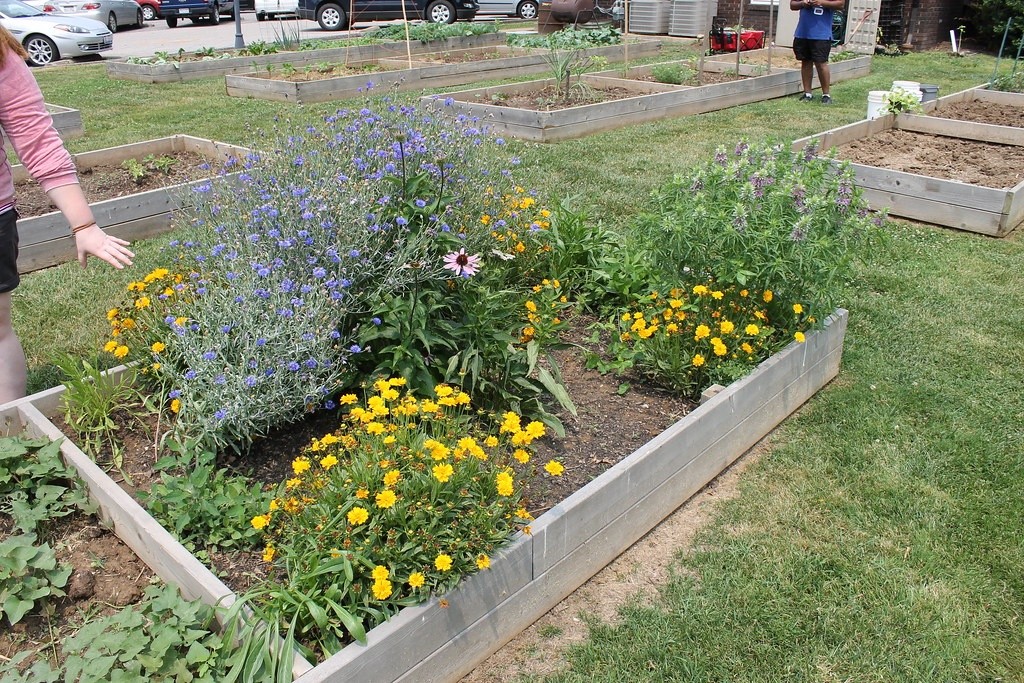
158,0,554,32
43,0,146,35
135,0,165,21
0,0,113,67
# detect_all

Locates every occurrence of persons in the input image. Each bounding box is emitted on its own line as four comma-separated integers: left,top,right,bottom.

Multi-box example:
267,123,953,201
790,0,845,104
0,23,135,407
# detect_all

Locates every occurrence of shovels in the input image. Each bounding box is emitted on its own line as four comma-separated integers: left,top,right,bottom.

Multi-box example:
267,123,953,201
836,10,872,53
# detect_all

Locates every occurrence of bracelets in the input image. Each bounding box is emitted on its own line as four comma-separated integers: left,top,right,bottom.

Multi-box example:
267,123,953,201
72,220,96,233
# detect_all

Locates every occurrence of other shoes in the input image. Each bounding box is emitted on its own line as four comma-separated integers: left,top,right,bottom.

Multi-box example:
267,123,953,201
819,96,833,107
798,93,814,103
